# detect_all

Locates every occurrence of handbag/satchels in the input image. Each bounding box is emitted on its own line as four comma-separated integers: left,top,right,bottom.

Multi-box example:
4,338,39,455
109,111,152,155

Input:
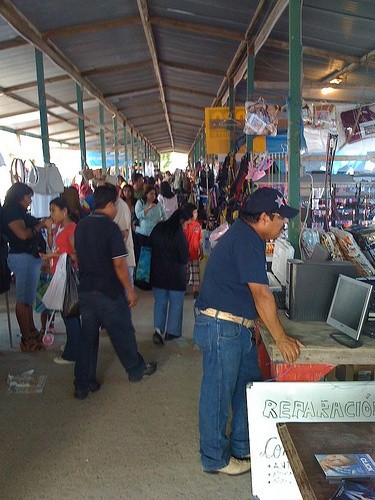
42,254,81,317
135,246,151,283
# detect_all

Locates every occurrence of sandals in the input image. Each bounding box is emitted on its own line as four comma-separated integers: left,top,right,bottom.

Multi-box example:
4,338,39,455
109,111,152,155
20,337,46,352
33,333,44,342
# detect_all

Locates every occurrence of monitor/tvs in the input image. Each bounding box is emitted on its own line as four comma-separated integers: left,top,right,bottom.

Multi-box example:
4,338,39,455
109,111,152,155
355,277,375,321
327,274,374,348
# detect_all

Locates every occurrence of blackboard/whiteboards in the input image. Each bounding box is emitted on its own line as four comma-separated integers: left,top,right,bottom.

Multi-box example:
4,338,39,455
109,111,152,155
246,381,374,500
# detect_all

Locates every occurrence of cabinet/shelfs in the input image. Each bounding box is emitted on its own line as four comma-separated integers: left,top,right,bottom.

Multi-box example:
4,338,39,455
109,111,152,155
253,172,375,258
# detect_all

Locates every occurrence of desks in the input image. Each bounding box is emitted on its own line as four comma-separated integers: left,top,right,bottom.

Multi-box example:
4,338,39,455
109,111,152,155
254,307,375,382
276,420,375,500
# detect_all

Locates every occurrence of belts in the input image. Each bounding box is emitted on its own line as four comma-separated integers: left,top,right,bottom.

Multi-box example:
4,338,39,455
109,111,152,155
200,307,252,328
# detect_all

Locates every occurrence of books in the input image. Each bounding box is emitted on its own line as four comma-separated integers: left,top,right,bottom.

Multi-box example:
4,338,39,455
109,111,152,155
313,454,375,500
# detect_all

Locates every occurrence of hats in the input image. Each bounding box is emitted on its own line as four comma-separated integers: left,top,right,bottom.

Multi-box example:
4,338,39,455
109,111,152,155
247,187,300,218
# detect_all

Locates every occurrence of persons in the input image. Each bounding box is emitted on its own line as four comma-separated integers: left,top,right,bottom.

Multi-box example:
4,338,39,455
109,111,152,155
147,207,193,346
73,185,158,400
192,186,306,476
317,454,356,472
1,182,49,353
35,169,204,305
38,196,81,365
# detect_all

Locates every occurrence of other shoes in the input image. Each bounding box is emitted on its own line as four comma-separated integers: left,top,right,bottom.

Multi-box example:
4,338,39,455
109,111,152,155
193,292,198,298
128,362,157,383
153,332,164,346
164,333,177,340
203,455,251,475
74,383,101,399
53,355,75,364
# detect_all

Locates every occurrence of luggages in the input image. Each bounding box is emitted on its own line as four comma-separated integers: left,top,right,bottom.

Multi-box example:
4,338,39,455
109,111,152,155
299,224,375,278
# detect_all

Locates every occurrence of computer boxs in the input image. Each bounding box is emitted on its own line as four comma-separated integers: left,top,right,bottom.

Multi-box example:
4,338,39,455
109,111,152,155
284,259,355,322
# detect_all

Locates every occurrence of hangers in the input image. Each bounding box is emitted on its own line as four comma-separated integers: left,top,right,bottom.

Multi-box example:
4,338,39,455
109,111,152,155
218,112,242,127
